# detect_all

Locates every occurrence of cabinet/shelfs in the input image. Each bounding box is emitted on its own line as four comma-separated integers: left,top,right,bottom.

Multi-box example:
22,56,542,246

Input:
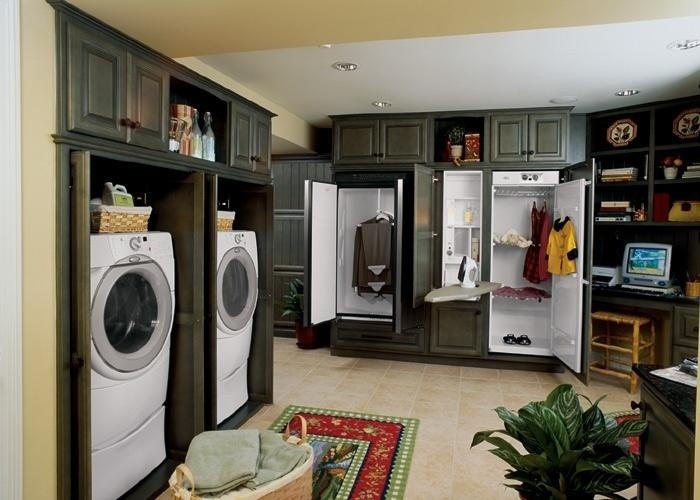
631,377,696,500
210,91,278,184
303,105,598,388
585,94,700,375
21,0,170,166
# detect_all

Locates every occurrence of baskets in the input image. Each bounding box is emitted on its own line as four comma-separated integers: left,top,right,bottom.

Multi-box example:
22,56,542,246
218,211,235,230
169,415,313,499
91,204,152,232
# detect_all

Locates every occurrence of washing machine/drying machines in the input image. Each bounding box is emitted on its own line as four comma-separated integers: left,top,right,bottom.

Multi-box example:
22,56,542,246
91,229,176,500
217,228,261,428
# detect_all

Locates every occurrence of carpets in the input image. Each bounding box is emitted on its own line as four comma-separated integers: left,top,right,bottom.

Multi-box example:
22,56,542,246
264,405,420,499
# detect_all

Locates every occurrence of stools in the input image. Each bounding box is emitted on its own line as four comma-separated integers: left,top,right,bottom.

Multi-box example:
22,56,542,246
587,308,671,395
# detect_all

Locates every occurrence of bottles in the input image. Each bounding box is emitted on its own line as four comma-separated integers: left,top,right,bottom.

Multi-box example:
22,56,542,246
201,112,216,161
463,201,474,227
187,108,203,160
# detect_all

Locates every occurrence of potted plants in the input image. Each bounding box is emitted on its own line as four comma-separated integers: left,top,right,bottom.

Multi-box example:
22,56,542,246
278,277,326,351
448,123,467,159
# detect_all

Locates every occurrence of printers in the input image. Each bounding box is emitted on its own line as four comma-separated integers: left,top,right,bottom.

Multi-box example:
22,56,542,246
591,266,621,287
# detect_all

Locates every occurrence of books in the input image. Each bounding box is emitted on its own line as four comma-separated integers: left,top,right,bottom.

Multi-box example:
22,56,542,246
600,167,639,184
594,200,633,223
682,163,700,178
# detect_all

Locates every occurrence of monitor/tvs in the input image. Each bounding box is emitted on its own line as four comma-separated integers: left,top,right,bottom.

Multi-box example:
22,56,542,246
621,243,672,288
